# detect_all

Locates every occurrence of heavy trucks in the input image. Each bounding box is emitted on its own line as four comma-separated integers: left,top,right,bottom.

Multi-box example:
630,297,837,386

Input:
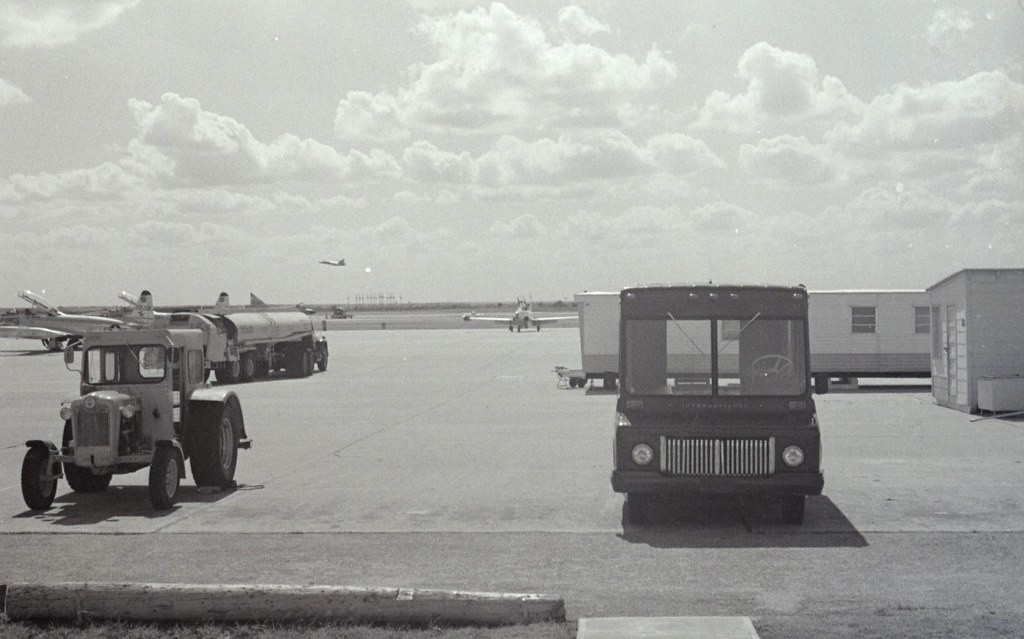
140,307,331,387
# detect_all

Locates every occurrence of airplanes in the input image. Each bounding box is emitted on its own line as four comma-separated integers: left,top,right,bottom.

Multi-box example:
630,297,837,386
0,290,130,356
460,293,582,332
319,259,348,269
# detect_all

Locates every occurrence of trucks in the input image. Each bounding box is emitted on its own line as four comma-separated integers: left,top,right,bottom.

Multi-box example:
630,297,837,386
608,282,825,529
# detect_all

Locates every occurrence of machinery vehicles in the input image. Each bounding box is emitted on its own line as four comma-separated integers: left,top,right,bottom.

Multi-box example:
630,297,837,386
21,324,253,515
